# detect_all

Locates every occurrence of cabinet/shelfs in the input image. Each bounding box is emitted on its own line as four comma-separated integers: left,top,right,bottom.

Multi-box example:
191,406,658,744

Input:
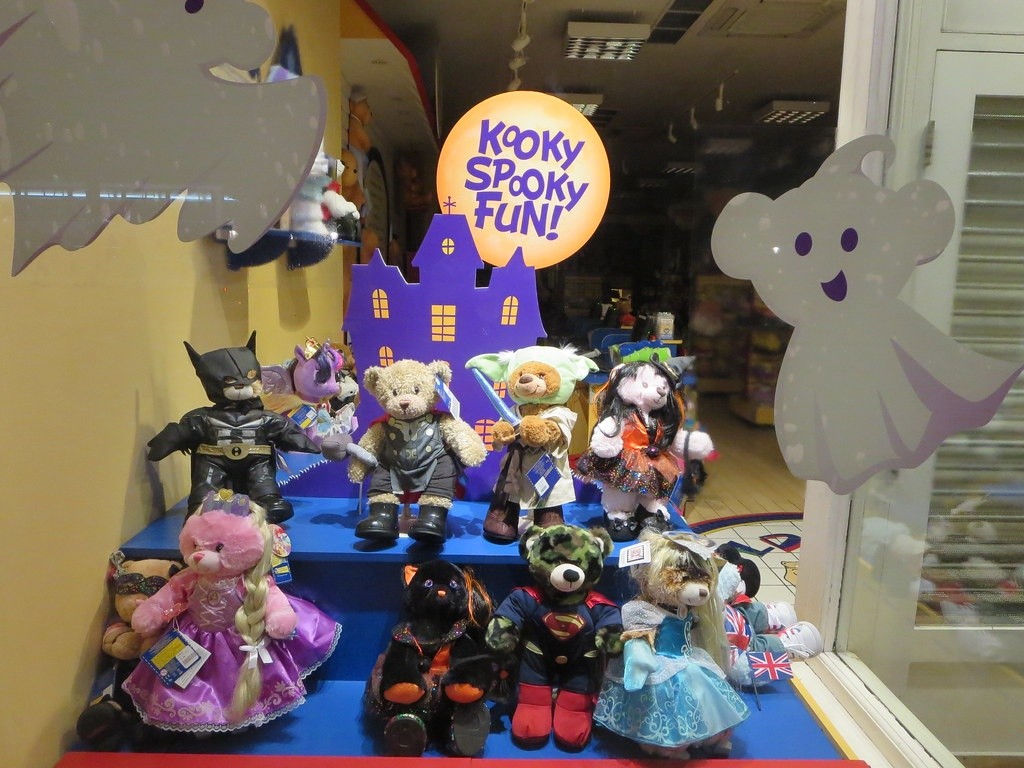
688,275,794,427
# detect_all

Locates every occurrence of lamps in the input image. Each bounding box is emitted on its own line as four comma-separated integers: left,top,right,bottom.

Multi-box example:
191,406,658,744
750,100,832,128
638,178,670,191
561,20,651,60
549,92,604,118
661,161,705,176
702,137,749,156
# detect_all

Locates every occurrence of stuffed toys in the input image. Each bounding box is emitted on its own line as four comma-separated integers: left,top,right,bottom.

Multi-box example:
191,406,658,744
74,551,190,753
707,541,827,692
254,334,362,462
361,559,507,755
121,488,343,740
463,344,599,543
916,514,1024,625
143,330,320,529
591,526,751,761
484,521,627,751
223,63,377,272
342,360,489,546
570,351,714,542
